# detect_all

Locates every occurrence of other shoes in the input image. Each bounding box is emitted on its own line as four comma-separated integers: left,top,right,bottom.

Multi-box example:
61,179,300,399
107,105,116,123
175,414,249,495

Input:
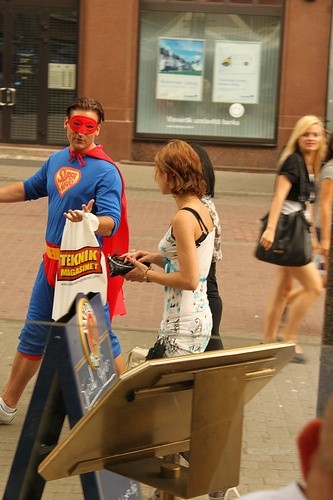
0,396,18,424
280,338,305,363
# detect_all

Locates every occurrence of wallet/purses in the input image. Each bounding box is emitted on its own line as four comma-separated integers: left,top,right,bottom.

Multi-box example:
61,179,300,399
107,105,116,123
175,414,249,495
107,253,151,276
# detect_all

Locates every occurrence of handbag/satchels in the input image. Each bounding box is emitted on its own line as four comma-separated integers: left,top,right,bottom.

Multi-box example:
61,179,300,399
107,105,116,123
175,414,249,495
51,209,108,323
255,154,312,268
